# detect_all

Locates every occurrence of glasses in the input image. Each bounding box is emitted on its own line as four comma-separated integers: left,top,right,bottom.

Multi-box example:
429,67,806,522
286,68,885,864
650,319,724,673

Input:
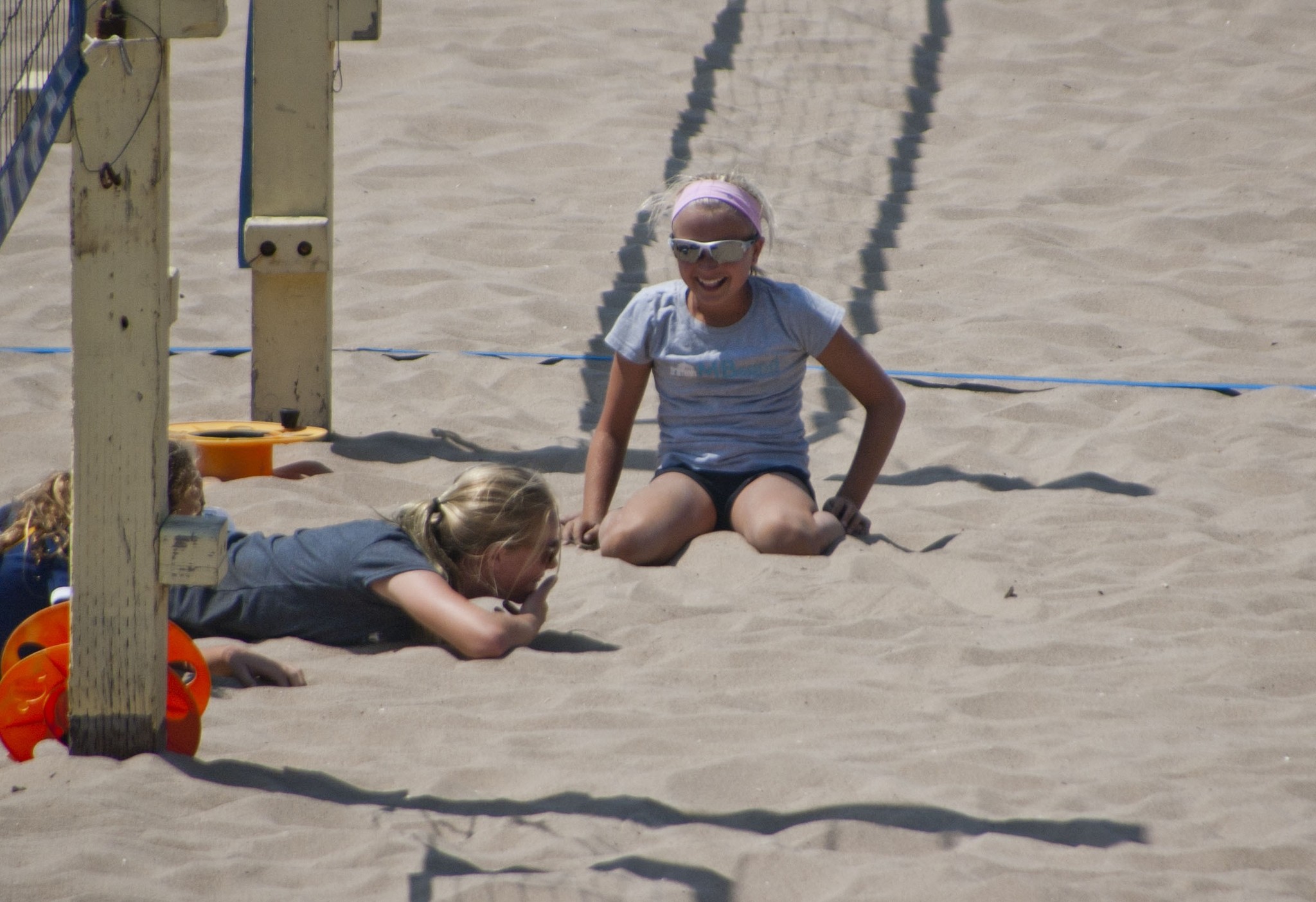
668,232,758,266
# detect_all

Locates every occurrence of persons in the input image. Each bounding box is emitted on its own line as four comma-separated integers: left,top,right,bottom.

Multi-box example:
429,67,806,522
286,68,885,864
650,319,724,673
0,441,561,688
559,173,907,565
